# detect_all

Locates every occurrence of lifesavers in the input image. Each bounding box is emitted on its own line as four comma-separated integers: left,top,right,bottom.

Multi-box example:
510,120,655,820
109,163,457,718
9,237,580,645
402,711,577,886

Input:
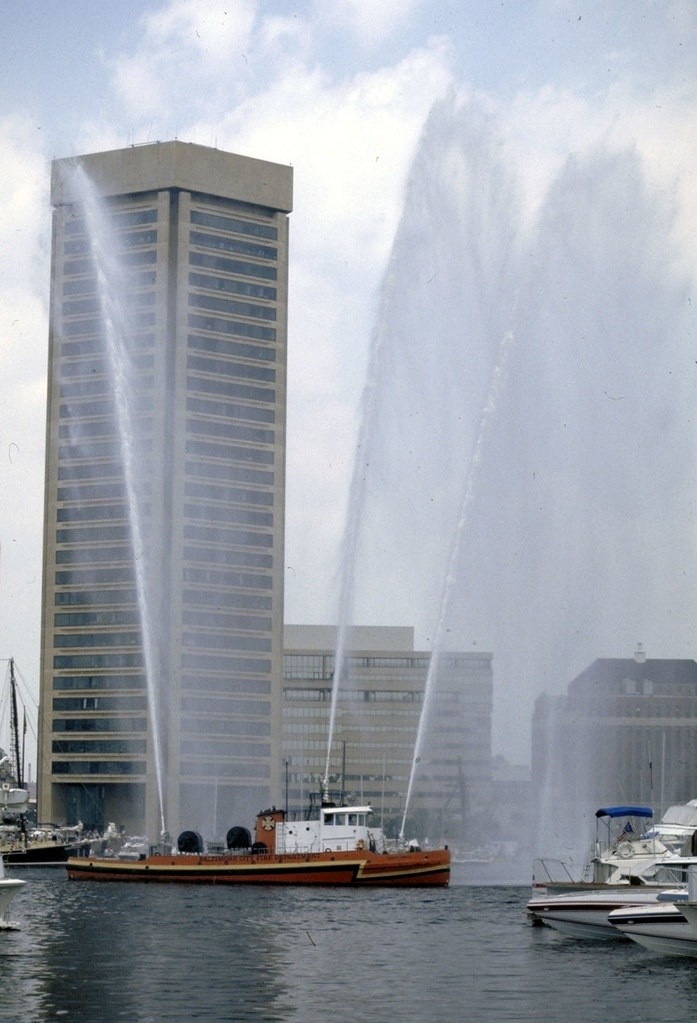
357,839,366,850
618,843,635,859
325,848,331,852
155,851,160,856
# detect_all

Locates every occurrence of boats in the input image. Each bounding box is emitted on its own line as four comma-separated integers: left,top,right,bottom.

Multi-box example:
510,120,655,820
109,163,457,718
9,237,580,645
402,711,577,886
526,758,697,959
66,740,452,887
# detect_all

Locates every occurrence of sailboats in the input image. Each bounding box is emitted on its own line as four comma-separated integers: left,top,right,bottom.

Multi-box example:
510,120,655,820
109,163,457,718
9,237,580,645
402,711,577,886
0,657,129,867
